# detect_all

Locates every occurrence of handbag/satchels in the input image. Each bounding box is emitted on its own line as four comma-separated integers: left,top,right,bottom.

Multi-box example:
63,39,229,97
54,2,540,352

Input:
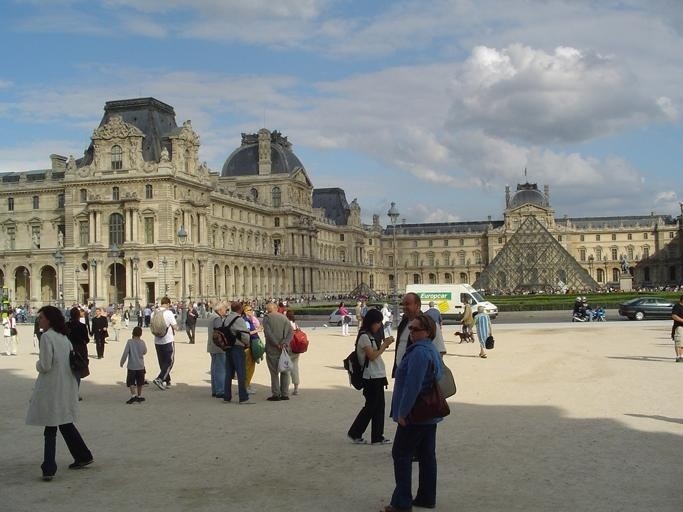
409,384,450,422
10,328,16,335
69,350,87,370
438,359,456,399
80,351,90,377
3,326,10,337
33,337,37,348
277,348,293,372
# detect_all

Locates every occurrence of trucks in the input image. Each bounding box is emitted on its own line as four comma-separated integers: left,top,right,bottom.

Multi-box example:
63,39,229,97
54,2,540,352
405,284,497,324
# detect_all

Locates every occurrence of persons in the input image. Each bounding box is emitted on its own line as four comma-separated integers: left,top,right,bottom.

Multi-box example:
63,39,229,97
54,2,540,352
2,303,37,357
619,254,631,274
572,296,582,317
582,296,593,316
619,255,626,272
204,293,306,403
108,296,199,405
391,292,446,462
32,232,39,246
25,305,94,482
670,297,683,363
57,300,132,359
67,154,78,172
495,285,682,298
160,146,169,161
347,308,396,446
310,288,494,360
57,231,65,248
379,313,444,511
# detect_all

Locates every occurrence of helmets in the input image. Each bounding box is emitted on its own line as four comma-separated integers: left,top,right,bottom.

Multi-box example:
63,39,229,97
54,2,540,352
576,297,581,301
581,296,587,302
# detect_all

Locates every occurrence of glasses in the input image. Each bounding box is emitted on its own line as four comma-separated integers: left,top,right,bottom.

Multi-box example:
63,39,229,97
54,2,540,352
38,315,42,320
247,309,252,311
408,324,425,332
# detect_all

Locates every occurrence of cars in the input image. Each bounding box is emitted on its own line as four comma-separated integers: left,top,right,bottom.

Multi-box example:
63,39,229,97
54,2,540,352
330,307,368,326
618,297,679,321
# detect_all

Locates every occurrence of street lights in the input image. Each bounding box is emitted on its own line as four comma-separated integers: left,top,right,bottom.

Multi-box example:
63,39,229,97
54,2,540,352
386,202,401,330
176,225,190,331
110,242,120,307
54,248,97,309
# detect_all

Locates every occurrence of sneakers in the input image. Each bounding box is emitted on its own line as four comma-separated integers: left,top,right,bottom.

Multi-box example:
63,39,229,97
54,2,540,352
136,397,145,402
68,459,96,470
679,357,683,363
153,377,174,387
351,438,368,444
371,435,393,445
210,387,303,405
42,473,57,482
676,358,679,362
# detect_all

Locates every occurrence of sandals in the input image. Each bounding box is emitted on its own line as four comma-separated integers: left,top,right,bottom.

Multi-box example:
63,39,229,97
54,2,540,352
412,495,435,509
379,504,413,512
481,354,487,358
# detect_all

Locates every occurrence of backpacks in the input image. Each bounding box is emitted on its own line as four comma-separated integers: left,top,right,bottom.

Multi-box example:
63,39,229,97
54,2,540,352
150,308,171,338
485,336,494,349
342,332,373,390
212,315,242,351
289,328,309,354
251,338,265,364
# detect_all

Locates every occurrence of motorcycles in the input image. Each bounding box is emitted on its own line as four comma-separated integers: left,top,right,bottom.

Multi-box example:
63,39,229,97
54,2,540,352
573,307,607,321
